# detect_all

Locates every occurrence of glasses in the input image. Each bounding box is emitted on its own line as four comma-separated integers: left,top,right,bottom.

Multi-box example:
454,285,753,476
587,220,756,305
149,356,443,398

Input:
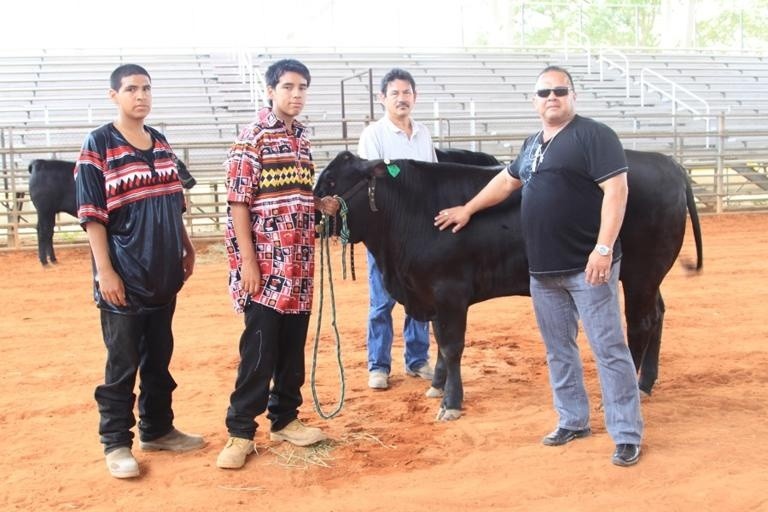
535,89,574,97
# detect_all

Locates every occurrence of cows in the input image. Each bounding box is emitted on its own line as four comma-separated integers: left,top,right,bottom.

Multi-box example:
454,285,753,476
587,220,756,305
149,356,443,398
315,150,703,422
29,158,77,266
435,148,501,166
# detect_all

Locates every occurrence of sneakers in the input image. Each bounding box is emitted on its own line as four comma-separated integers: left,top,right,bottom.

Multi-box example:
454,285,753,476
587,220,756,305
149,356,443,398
105,446,140,478
216,436,255,469
407,364,435,380
139,428,205,451
612,444,642,465
543,427,590,444
369,370,388,388
270,418,323,445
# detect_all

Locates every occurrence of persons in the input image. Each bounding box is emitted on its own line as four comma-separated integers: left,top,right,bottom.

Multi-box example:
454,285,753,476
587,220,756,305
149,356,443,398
434,66,644,467
74,64,205,479
216,60,338,469
359,68,439,389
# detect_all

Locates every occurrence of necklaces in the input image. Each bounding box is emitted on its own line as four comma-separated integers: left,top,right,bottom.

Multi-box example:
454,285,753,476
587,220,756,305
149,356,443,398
529,120,572,173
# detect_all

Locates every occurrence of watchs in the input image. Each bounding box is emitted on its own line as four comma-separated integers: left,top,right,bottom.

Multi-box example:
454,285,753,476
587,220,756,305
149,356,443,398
595,245,613,256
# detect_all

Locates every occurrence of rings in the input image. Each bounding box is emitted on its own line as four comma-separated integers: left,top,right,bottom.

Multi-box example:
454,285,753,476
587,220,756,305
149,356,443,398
444,212,448,216
599,273,605,277
